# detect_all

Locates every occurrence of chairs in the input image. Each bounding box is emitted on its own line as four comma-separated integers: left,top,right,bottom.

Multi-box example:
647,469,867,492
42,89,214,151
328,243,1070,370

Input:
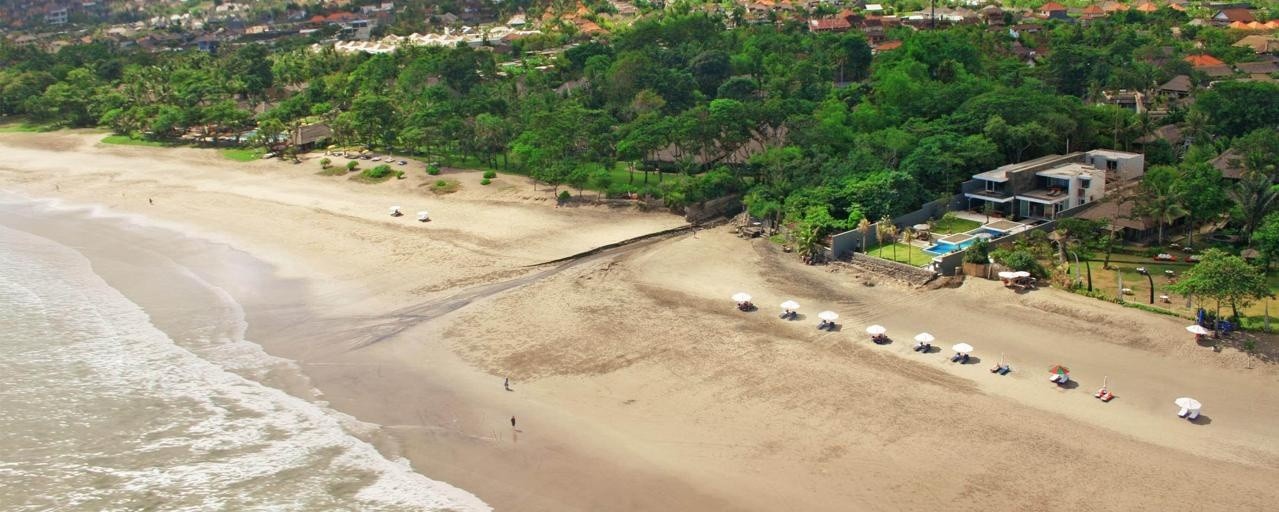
1049,374,1061,382
1058,374,1069,384
1188,408,1200,420
914,342,1009,375
781,309,834,331
1177,408,1189,417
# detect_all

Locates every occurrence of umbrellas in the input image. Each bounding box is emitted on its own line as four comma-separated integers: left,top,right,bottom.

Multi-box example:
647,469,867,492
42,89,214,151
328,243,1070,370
818,309,840,322
730,292,752,303
781,299,802,311
951,342,977,354
865,324,888,336
915,330,936,343
1046,364,1072,375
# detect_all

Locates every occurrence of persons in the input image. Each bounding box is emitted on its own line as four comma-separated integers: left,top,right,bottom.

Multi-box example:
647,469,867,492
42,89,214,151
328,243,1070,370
504,377,510,388
510,416,516,430
